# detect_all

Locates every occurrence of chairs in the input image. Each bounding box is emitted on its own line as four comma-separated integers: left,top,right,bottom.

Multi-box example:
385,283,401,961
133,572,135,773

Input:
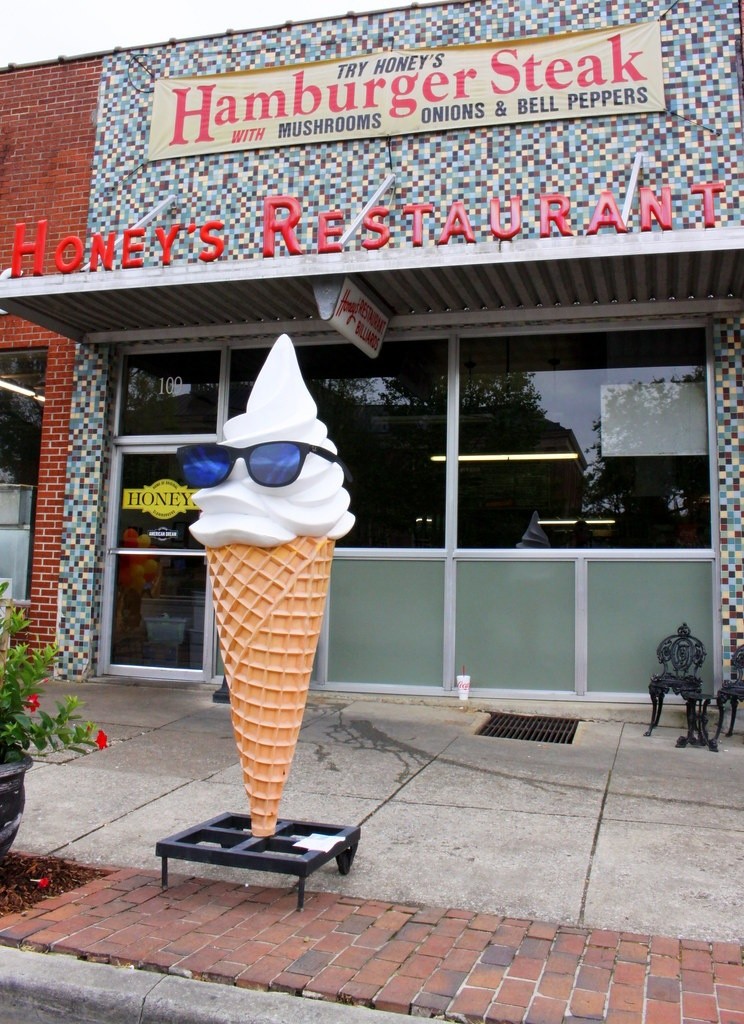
643,622,707,737
715,645,744,739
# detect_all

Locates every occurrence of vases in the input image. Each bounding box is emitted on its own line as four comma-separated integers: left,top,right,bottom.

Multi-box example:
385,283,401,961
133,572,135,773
0,749,35,862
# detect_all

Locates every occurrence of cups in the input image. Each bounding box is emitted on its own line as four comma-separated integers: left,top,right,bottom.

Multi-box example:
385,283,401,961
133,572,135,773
456,675,470,701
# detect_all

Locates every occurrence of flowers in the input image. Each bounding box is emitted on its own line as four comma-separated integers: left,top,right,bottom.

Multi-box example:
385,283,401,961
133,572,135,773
0,580,107,766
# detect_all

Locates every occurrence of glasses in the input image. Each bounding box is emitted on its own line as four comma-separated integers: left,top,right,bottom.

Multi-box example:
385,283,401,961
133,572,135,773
176,441,354,489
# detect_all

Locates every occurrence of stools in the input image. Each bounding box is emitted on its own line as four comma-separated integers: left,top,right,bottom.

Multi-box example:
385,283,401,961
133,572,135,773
676,694,719,752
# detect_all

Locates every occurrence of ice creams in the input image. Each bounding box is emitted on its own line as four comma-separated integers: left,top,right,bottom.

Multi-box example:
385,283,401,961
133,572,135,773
188,332,357,838
